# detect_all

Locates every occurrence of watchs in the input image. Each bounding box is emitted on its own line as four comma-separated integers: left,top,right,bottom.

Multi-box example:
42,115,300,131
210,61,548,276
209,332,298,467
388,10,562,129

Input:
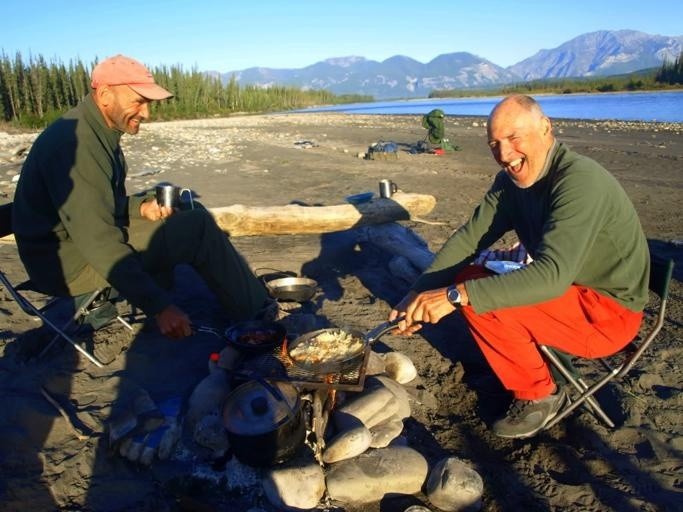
447,284,463,309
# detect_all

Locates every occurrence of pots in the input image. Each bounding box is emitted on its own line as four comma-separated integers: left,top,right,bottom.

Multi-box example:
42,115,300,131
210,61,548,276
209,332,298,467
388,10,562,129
255,268,317,302
288,315,413,374
219,367,306,467
189,320,286,352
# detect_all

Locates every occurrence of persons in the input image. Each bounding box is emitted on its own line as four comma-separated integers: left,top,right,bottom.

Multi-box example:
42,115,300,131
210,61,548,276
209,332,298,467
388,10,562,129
389,94,651,438
11,53,316,342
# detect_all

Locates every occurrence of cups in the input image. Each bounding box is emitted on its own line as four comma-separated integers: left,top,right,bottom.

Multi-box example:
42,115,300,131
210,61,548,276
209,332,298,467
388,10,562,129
379,179,397,199
156,185,178,208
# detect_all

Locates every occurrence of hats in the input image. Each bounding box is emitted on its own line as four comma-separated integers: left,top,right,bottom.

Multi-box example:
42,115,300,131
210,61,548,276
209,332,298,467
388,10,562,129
91,55,173,101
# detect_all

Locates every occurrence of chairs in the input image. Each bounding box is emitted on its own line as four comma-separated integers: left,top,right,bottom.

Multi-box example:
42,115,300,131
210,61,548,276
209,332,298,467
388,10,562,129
0,202,136,369
539,256,675,430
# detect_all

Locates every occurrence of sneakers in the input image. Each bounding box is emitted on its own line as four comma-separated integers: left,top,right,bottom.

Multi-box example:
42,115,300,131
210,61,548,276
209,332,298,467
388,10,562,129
494,383,572,437
274,301,315,332
